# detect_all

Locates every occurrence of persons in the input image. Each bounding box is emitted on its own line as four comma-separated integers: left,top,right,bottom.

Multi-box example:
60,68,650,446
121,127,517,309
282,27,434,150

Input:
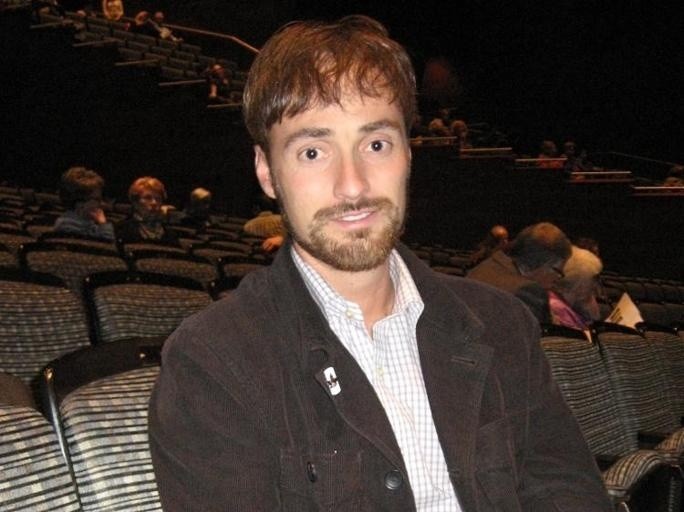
37,2,231,104
147,11,615,511
461,220,605,330
51,165,285,254
409,105,684,186
